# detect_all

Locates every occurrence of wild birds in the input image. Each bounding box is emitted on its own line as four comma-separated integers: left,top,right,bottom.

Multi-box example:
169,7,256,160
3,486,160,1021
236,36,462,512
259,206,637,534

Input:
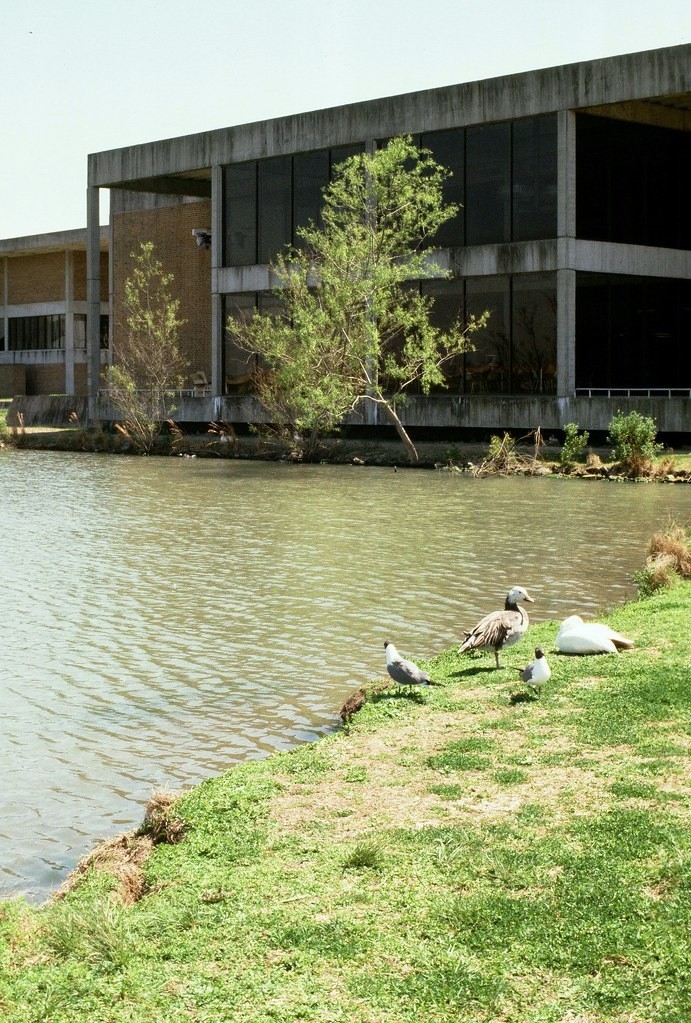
457,587,535,669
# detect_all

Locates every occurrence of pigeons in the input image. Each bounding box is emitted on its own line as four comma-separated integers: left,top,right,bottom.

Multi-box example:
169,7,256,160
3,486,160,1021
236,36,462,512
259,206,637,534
383,640,446,691
556,615,634,654
510,647,552,700
